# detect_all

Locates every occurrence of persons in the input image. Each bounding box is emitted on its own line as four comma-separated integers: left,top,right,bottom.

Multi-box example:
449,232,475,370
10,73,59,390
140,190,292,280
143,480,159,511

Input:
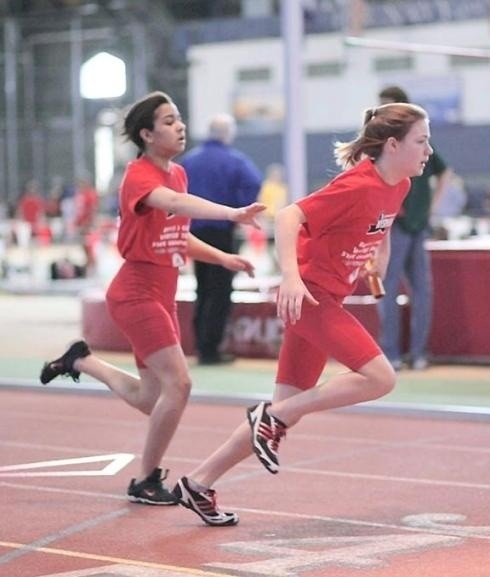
169,102,434,526
257,162,288,222
375,85,451,372
13,169,125,282
175,114,268,365
39,90,268,505
423,160,469,240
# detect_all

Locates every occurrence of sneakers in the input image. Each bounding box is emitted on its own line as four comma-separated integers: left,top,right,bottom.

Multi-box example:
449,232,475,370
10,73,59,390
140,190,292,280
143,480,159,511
38,337,91,387
246,401,290,476
372,347,435,373
124,468,179,509
172,477,241,528
193,347,239,366
